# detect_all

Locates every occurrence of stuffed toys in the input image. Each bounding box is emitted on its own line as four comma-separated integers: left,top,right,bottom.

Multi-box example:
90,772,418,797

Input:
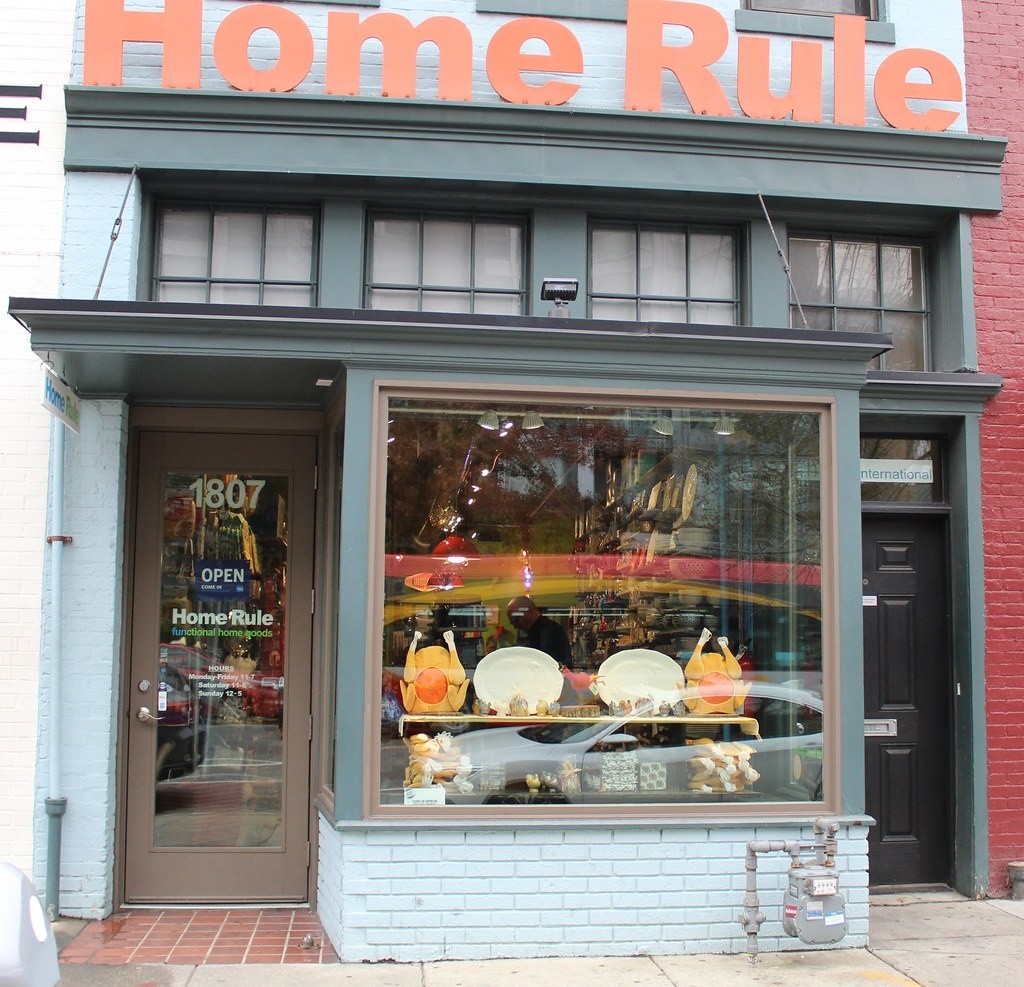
403,733,474,795
677,627,752,715
400,629,469,713
686,736,759,793
558,664,605,691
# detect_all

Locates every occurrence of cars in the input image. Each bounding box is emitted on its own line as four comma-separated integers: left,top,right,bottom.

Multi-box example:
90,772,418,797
433,680,825,808
157,639,278,723
151,682,212,785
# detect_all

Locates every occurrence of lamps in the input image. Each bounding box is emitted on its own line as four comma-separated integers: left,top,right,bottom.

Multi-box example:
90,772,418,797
477,410,499,430
713,418,736,435
502,419,513,429
540,277,579,318
521,410,545,430
652,415,674,435
498,429,508,437
459,497,474,505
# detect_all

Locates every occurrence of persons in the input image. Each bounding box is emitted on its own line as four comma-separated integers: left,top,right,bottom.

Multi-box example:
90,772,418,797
508,595,573,671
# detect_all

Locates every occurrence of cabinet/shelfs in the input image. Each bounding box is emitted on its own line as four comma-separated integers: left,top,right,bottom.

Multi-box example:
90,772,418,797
602,444,720,640
398,714,763,801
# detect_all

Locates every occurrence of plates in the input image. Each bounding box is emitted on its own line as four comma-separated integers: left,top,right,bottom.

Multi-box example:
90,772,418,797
681,463,697,521
646,527,658,563
597,649,684,716
671,485,680,507
663,473,675,508
473,647,564,714
648,481,662,509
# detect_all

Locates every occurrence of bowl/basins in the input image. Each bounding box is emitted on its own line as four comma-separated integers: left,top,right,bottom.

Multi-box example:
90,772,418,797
678,527,713,549
637,587,723,629
